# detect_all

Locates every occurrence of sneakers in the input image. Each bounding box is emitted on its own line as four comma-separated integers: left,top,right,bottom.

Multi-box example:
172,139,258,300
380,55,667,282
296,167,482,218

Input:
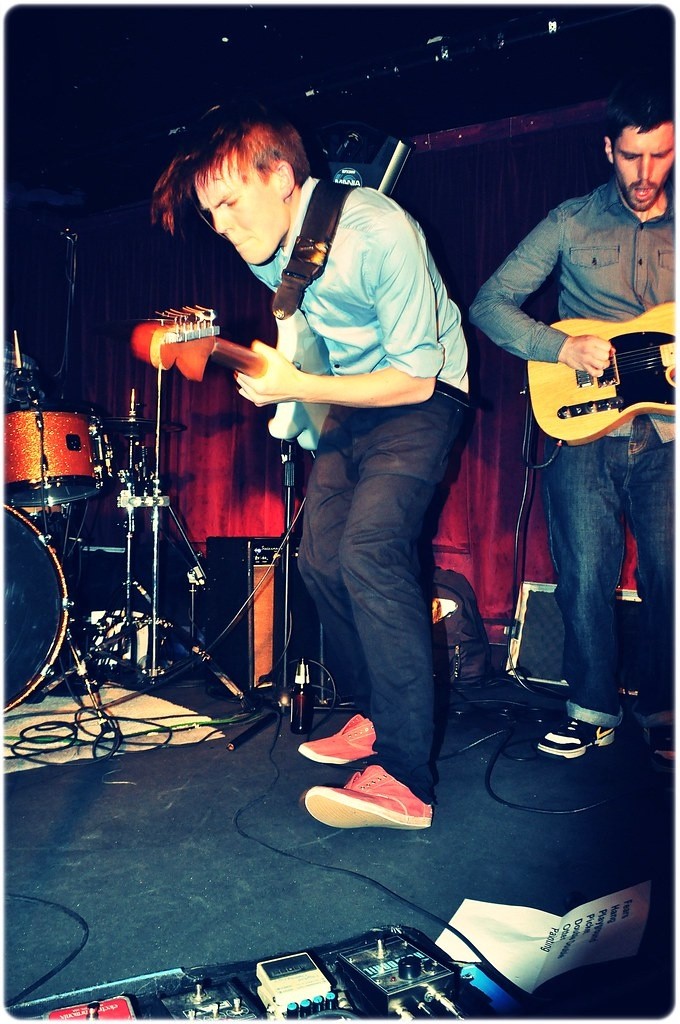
298,714,378,764
304,765,433,830
641,721,675,770
537,719,614,758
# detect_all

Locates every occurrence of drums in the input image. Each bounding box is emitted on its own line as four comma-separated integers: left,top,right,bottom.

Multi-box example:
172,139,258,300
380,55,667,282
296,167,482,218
4,398,102,507
6,503,70,711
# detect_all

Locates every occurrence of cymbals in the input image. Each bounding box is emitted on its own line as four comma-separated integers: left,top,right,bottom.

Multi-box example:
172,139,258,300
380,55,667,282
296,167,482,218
105,414,157,432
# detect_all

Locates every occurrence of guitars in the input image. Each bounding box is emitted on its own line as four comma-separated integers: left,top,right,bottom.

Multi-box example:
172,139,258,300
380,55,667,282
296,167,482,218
527,301,675,445
133,302,328,450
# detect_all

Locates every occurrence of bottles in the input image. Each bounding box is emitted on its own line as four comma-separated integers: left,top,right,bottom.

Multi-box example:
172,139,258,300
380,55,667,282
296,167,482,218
289,657,315,734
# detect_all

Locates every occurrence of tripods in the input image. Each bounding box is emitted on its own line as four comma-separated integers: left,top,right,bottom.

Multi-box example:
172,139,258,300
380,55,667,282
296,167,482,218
23,368,363,752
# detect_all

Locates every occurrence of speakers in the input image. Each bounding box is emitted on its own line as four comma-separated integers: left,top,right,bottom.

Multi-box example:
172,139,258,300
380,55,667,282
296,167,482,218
202,535,282,697
504,582,641,699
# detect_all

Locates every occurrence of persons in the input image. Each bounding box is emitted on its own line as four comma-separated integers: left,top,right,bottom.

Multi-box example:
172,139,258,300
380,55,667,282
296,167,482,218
465,79,676,765
152,102,468,836
